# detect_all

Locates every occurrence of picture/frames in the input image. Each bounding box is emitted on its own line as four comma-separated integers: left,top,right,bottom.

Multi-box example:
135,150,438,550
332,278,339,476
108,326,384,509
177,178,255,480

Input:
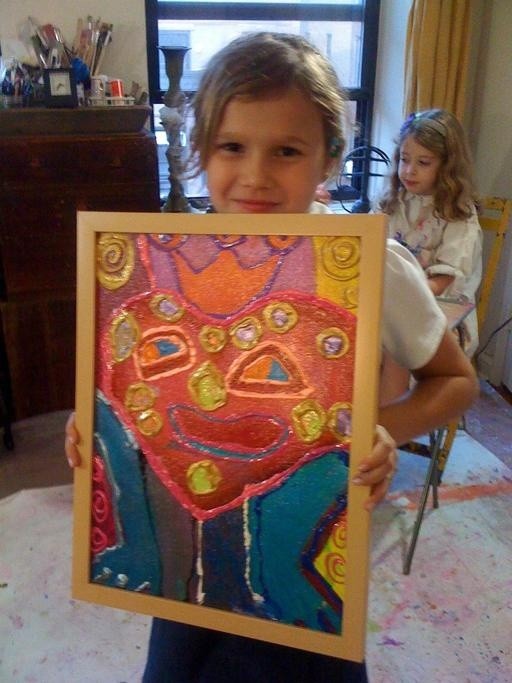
74,210,388,663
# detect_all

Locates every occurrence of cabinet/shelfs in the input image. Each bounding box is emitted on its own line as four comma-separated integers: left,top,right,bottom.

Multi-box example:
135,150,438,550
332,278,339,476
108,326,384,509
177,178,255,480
1,133,163,424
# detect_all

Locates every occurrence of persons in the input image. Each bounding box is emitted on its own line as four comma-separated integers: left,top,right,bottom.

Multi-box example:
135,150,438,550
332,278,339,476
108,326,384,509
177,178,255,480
66,28,481,683
366,108,482,408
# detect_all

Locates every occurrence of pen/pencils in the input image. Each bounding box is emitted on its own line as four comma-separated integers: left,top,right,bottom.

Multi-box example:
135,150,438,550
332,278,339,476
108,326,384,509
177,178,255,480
27,16,69,69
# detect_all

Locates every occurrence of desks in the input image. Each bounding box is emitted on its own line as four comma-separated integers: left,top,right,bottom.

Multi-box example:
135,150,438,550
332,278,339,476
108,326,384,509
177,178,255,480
397,298,476,576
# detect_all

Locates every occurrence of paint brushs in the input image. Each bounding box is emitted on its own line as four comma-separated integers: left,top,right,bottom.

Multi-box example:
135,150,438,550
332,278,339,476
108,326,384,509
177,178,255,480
72,15,114,78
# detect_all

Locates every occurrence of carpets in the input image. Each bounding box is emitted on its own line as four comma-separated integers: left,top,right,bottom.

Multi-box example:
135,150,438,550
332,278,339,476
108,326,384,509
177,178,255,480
1,427,512,682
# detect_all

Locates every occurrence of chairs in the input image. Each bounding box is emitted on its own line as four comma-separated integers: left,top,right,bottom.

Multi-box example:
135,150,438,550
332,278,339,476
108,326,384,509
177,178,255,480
394,195,509,486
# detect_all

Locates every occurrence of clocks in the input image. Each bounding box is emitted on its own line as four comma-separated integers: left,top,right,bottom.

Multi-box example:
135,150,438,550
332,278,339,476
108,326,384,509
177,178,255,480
44,67,79,108
